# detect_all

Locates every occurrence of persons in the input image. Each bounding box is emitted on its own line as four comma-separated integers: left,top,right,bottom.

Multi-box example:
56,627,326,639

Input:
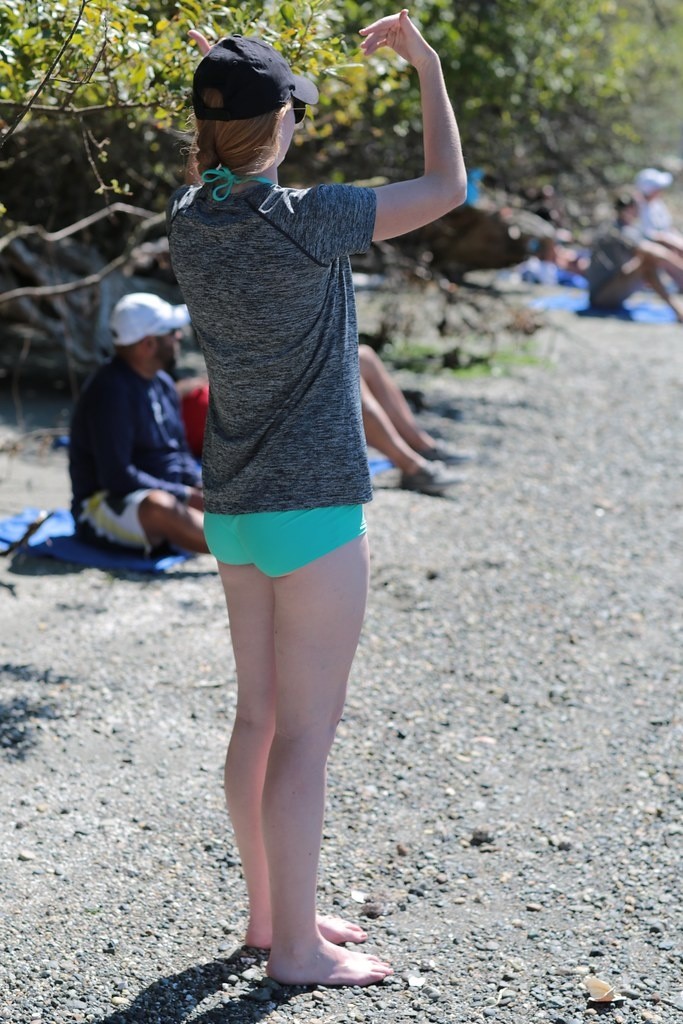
67,291,211,554
175,3,469,986
585,191,683,324
169,344,472,491
635,167,678,238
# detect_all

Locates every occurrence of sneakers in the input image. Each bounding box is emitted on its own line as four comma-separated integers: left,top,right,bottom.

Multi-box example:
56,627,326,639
415,437,473,466
399,458,463,497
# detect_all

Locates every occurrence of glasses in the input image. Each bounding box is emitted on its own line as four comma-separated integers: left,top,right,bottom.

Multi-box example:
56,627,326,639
288,98,307,124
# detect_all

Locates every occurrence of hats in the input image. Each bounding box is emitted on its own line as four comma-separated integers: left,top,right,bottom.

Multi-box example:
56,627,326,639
191,33,320,122
108,291,192,347
635,167,673,197
